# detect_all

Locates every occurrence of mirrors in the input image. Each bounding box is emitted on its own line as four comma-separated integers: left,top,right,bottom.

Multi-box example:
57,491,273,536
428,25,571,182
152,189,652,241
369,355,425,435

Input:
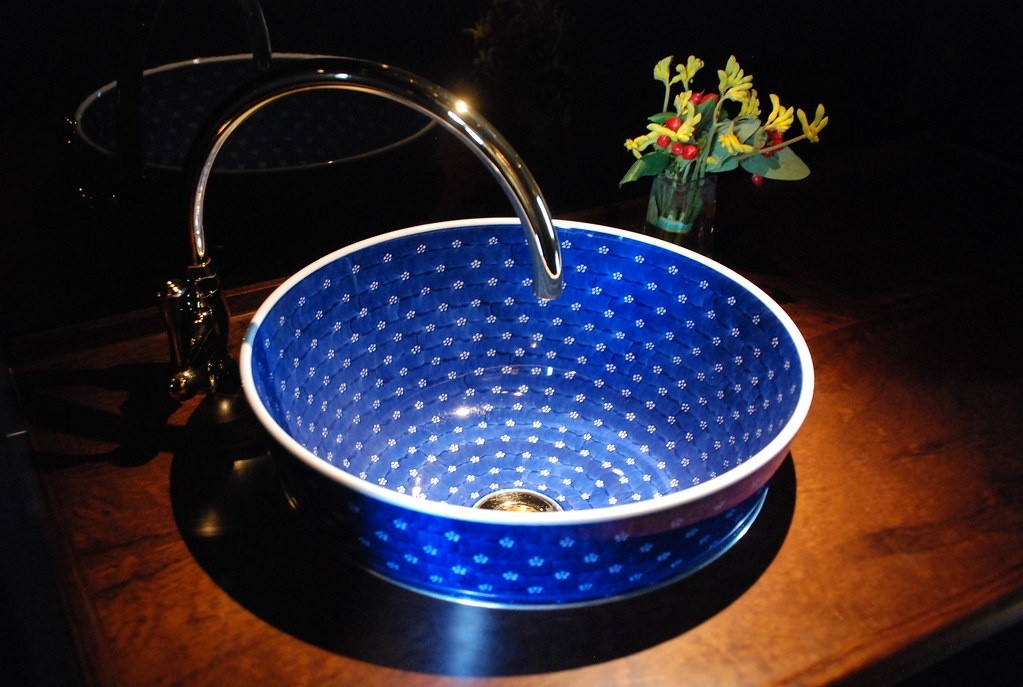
0,0,936,344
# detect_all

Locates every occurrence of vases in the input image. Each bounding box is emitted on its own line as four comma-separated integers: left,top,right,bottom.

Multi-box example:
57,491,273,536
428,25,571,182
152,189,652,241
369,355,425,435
642,171,718,262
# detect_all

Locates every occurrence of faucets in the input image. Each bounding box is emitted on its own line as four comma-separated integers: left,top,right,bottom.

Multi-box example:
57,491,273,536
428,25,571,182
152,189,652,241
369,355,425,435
155,70,563,425
232,0,276,86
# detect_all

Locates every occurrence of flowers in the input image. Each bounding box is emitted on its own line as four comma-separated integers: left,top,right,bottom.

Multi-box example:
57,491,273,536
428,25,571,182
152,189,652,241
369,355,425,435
461,1,579,69
619,53,830,222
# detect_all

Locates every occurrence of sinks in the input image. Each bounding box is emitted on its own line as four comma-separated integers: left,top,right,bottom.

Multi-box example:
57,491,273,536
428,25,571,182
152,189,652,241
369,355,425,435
72,50,439,226
238,216,815,613
